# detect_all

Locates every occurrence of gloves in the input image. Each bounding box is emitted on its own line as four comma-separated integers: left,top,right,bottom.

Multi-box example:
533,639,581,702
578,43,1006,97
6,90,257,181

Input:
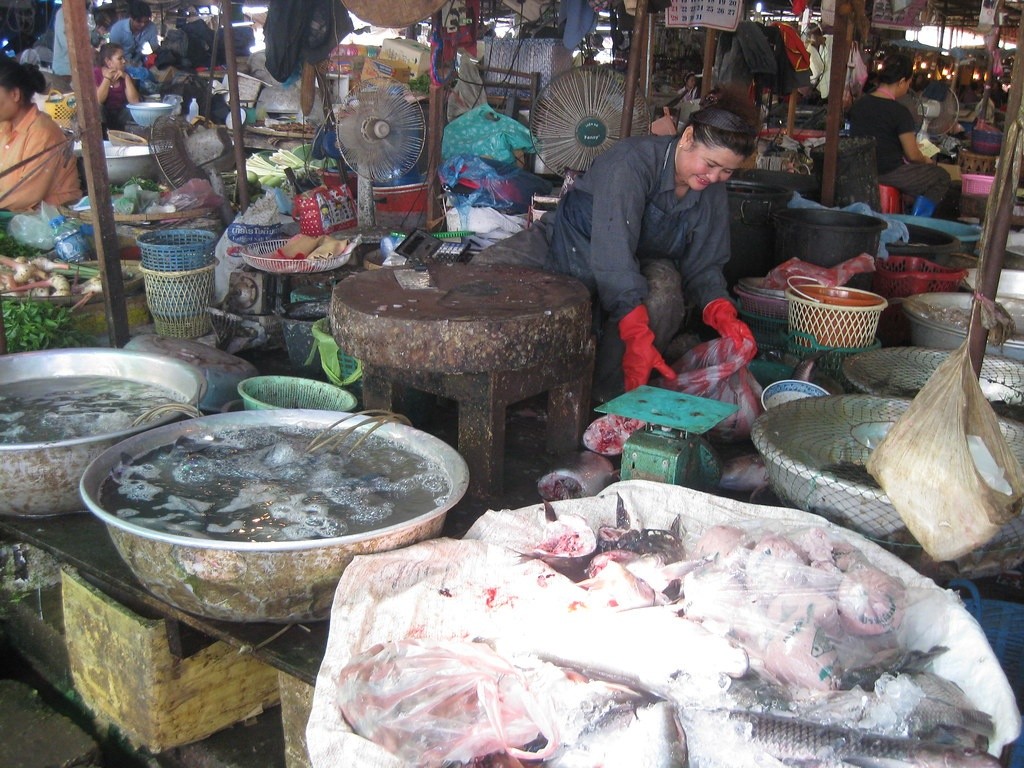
701,298,759,363
618,303,676,393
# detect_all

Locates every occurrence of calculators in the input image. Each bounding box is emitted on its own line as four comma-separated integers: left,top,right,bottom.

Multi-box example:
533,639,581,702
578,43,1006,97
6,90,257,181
393,229,470,263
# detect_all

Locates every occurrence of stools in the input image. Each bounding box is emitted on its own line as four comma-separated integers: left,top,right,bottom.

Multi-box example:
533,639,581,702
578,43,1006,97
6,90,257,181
877,184,900,216
361,333,598,500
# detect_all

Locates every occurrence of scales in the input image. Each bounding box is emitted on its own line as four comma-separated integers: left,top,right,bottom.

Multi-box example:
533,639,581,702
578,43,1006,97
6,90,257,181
593,386,741,489
225,100,264,130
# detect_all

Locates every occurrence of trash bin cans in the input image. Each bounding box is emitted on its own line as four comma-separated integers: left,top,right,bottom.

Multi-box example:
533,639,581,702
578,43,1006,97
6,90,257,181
133,227,220,342
311,316,363,410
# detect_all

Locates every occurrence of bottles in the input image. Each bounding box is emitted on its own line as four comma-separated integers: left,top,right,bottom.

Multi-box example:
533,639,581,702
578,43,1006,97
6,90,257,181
50,215,91,262
189,98,199,123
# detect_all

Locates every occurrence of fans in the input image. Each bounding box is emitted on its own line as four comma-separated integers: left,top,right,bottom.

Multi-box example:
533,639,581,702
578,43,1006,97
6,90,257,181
147,115,235,228
914,83,960,142
331,73,427,244
529,61,654,179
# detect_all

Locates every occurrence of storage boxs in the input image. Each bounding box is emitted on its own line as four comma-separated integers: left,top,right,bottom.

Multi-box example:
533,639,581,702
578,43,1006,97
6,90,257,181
222,74,262,109
58,563,315,768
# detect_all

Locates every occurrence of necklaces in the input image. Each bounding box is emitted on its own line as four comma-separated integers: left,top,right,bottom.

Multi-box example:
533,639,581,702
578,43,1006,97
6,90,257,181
877,88,895,101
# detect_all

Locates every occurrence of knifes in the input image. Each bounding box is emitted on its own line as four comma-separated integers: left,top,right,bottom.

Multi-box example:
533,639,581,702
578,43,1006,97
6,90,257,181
394,259,436,292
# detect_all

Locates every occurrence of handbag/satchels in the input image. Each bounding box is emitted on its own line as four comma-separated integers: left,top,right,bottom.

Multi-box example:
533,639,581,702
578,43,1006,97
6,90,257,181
45,90,77,124
866,333,1024,562
291,183,357,237
443,105,539,167
484,21,574,99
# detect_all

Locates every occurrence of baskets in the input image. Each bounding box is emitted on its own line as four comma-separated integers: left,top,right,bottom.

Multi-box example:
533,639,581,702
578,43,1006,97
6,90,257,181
238,375,359,412
0,260,145,310
312,317,363,398
134,228,218,318
237,239,353,275
138,259,222,339
960,173,996,196
873,254,969,346
734,276,888,359
339,0,450,30
56,204,215,229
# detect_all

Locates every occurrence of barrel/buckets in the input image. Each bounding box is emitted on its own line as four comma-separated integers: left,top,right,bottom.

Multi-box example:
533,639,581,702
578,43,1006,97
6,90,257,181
276,301,333,383
723,168,888,292
787,274,883,351
370,182,441,230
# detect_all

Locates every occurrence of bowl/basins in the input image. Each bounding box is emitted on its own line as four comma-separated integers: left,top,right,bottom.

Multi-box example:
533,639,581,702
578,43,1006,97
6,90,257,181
841,346,1024,406
903,291,1024,361
79,408,469,621
757,128,827,140
125,335,260,413
745,360,796,390
127,101,174,125
750,394,1024,577
761,380,830,411
106,145,168,185
0,347,207,518
961,267,1024,300
875,213,981,265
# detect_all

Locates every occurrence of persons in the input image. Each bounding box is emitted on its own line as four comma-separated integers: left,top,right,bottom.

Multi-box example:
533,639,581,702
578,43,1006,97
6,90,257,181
109,2,159,89
849,55,951,216
95,42,143,131
677,71,700,101
91,22,108,48
0,53,84,213
52,0,99,94
467,85,758,398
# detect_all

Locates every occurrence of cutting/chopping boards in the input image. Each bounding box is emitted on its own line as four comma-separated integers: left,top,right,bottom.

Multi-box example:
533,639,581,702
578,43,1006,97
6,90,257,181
331,261,593,375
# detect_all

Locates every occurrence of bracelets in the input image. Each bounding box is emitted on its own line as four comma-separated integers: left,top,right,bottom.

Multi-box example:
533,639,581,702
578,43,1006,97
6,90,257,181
104,76,111,81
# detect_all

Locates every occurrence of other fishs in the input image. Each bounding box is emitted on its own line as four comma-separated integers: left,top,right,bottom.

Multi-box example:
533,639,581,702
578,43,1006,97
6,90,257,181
111,433,390,528
369,492,1006,768
0,389,150,427
0,256,102,297
